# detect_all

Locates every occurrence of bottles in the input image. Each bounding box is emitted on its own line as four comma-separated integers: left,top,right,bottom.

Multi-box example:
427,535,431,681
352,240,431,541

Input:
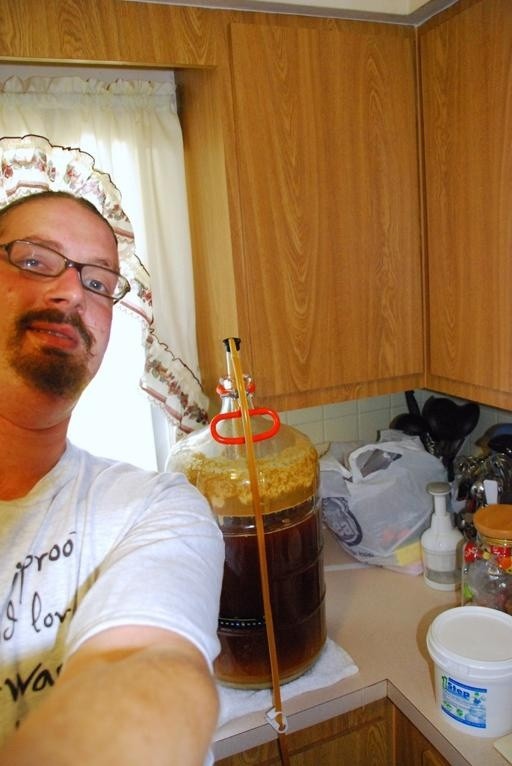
461,502,511,615
422,482,464,591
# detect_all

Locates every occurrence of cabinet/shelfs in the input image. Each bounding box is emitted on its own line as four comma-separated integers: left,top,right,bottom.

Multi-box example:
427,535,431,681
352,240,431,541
211,697,454,765
180,2,512,416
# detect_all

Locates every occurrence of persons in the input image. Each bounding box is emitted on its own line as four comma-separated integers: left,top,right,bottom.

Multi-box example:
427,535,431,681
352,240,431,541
0,187,230,766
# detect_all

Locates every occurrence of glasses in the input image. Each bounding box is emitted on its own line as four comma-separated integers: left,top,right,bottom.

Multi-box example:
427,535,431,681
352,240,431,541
0,238,130,304
